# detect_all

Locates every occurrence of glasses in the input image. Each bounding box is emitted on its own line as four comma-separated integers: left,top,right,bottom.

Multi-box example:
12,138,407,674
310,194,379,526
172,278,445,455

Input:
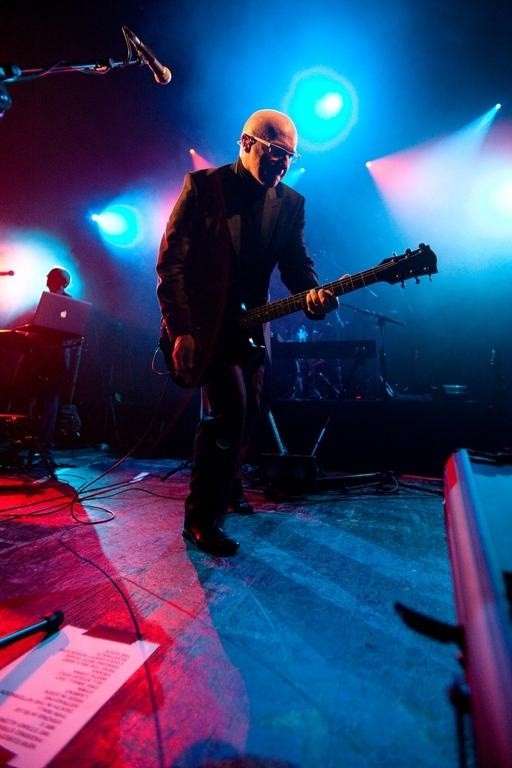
240,131,302,166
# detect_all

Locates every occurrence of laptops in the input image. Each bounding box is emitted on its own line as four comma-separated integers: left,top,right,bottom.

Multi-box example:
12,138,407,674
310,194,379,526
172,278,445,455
15,291,93,338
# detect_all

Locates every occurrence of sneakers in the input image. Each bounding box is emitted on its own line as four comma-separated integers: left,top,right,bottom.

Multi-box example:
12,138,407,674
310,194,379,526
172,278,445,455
182,520,241,555
230,485,254,513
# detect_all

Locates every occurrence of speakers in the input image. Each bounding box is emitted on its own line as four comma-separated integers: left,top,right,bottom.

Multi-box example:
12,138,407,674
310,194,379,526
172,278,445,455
271,339,377,398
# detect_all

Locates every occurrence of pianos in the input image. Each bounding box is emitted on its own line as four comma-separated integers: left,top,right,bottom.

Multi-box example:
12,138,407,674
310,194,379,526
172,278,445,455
272,340,375,359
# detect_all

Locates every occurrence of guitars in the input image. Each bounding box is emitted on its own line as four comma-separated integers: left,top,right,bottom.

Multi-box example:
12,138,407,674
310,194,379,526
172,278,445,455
160,242,438,387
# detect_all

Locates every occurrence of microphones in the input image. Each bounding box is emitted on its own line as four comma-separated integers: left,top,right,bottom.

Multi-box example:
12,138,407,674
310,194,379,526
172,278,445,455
121,25,172,85
0,270,14,276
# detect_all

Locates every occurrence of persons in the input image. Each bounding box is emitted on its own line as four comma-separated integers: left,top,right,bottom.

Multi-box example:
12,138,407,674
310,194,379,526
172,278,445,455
10,265,75,447
155,108,353,557
